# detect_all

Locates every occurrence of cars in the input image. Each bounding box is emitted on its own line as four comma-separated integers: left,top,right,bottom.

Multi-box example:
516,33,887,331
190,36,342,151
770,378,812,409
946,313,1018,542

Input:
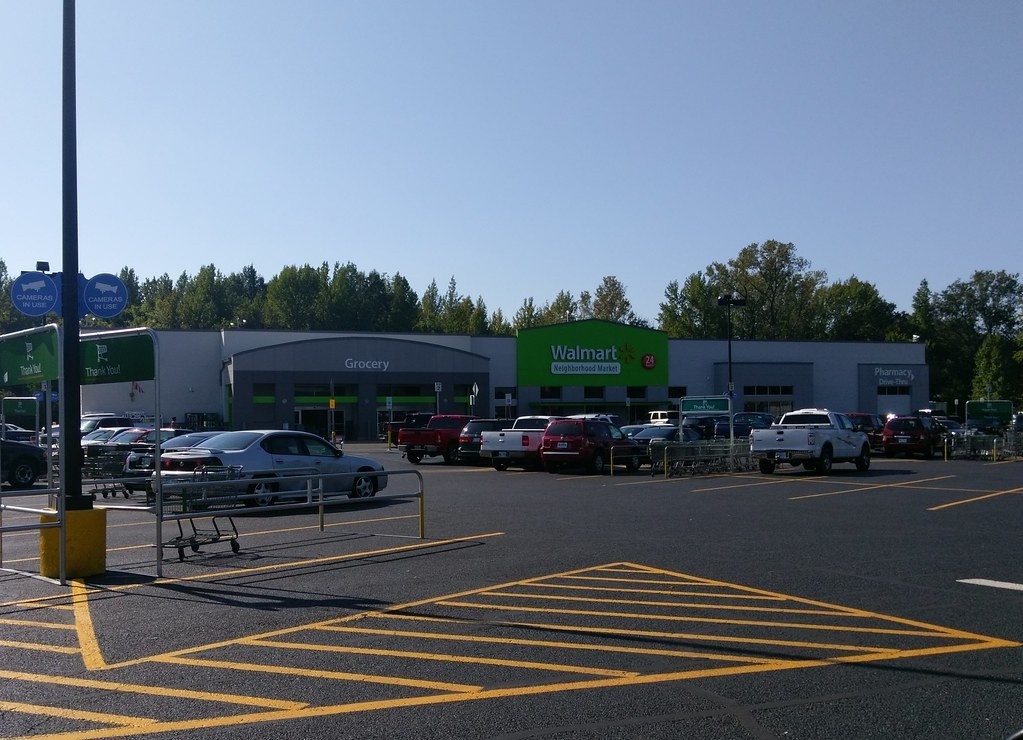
838,399,1009,460
2,369,769,526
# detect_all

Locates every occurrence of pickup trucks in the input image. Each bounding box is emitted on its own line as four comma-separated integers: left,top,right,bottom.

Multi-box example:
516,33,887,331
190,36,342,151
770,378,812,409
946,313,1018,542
746,406,872,475
